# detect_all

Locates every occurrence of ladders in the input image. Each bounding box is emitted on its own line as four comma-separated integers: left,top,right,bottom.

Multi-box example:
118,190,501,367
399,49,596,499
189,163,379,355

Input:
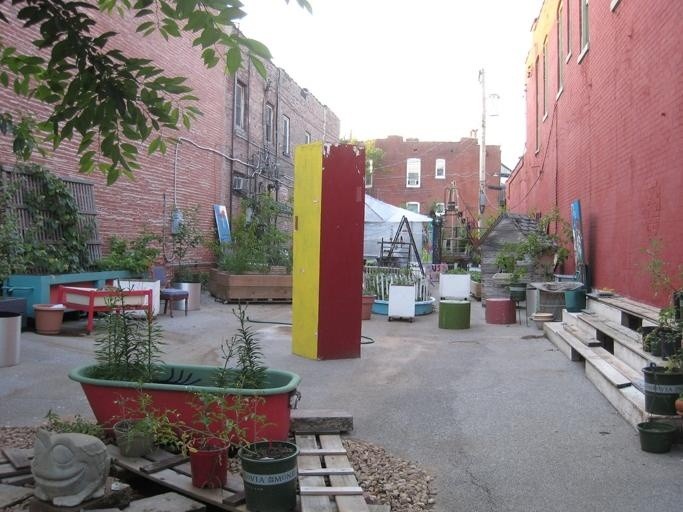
388,215,424,275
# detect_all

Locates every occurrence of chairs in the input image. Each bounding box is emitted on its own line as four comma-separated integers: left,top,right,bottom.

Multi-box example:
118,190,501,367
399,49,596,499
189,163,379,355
152,266,189,317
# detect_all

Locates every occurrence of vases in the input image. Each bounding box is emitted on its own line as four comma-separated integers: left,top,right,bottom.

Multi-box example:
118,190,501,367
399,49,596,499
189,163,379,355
33,303,66,335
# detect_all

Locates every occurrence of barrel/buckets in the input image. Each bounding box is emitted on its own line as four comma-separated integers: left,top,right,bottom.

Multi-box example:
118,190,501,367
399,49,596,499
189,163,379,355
644,366,683,415
638,423,677,454
565,290,585,313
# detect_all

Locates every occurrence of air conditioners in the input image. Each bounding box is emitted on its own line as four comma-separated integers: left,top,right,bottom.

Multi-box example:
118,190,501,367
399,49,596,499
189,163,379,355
232,177,248,192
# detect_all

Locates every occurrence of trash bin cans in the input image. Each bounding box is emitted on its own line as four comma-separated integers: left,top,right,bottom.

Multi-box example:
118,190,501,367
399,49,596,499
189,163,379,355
0,311,22,367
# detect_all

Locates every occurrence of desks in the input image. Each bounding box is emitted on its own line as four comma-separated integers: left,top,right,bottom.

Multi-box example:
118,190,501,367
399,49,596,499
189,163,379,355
58,284,153,334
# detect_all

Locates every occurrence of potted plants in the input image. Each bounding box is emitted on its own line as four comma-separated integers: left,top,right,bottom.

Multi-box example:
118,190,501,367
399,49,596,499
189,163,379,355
360,273,378,320
636,237,683,453
439,243,528,302
170,202,210,311
45,374,300,512
